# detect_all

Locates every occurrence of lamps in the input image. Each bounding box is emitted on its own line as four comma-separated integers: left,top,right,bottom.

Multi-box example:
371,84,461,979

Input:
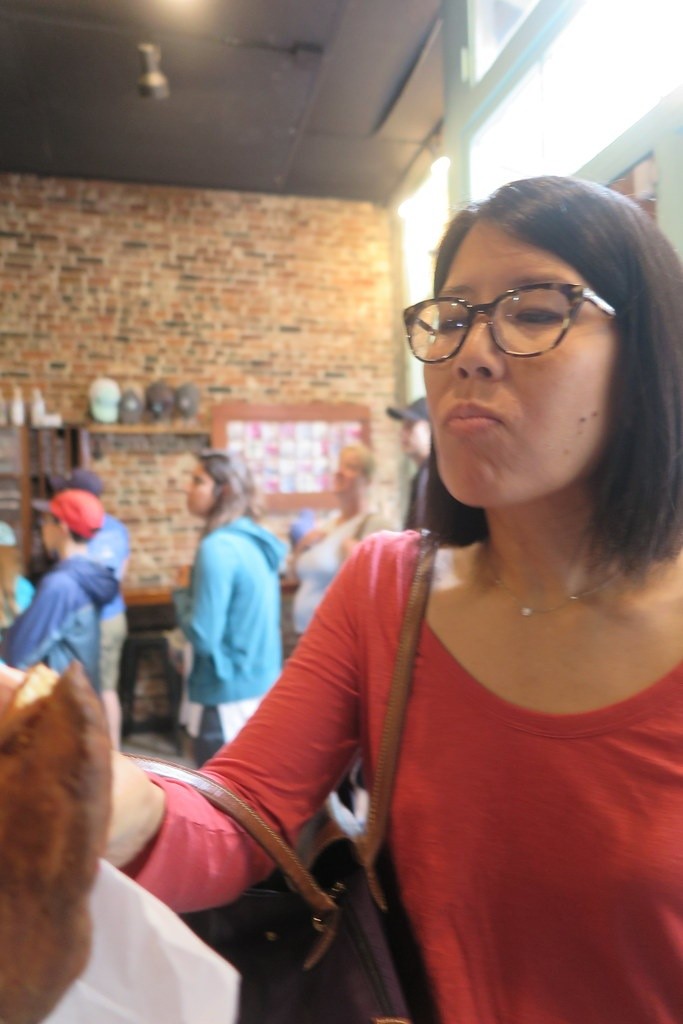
136,42,166,92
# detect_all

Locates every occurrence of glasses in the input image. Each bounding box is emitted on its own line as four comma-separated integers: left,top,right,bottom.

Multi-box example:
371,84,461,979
402,284,617,364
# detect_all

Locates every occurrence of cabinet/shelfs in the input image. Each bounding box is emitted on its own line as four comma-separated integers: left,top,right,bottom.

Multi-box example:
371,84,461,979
118,587,186,756
0,426,31,576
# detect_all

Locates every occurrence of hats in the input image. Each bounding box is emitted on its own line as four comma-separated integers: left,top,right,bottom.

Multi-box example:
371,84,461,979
31,485,106,541
387,396,431,423
50,470,104,498
85,377,200,423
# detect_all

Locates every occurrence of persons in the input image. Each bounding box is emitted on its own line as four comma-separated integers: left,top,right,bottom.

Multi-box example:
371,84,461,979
0,175,683,1024
6,469,131,749
166,447,287,767
294,394,434,643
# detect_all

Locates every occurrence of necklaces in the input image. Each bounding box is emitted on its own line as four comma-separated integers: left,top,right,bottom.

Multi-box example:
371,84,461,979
487,568,628,616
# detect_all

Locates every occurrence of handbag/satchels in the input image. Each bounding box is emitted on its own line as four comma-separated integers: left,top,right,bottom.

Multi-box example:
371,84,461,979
115,525,436,1024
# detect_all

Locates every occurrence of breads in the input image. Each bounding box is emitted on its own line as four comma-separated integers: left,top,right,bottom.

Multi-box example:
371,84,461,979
0,660,113,1024
